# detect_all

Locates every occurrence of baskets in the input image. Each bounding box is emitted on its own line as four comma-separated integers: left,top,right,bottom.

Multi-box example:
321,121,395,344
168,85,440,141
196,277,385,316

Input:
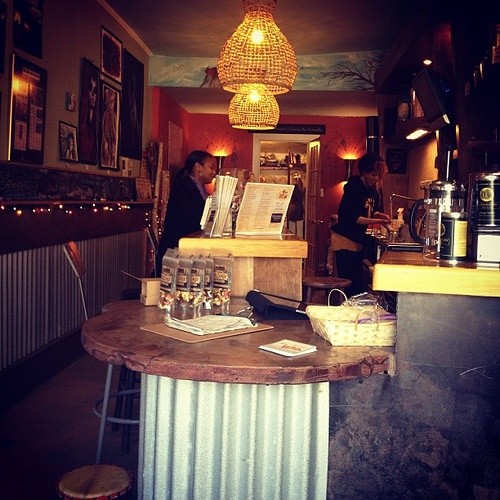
306,288,397,347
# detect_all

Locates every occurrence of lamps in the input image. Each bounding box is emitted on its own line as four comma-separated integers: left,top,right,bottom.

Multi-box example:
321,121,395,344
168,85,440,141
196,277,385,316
215,156,226,169
344,159,355,180
216,0,298,131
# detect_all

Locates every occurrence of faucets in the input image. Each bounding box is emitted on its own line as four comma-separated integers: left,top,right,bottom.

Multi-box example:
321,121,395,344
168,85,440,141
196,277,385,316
389,194,418,203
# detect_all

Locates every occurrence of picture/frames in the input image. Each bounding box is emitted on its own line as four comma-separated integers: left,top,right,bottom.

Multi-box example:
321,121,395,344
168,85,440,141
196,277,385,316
58,24,124,171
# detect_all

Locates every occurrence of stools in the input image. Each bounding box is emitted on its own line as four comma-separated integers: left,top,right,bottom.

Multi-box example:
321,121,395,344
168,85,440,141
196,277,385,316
302,277,351,305
63,240,140,464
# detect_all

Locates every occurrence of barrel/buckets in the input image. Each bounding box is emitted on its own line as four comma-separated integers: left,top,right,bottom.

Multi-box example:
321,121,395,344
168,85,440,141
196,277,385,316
420,181,467,261
467,172,500,231
436,212,470,264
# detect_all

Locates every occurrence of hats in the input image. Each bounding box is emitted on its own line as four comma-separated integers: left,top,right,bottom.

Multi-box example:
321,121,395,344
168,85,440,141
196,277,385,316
357,151,384,173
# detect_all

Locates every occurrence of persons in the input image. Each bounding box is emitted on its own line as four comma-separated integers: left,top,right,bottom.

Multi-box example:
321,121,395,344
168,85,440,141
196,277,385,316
156,151,218,277
330,155,395,297
